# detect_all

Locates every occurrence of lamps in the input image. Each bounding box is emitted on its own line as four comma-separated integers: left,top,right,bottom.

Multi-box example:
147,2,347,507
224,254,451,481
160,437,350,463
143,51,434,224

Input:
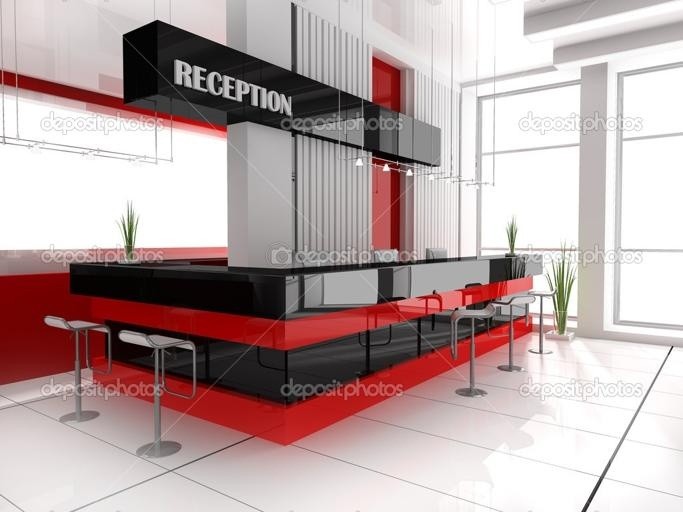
334,0,497,187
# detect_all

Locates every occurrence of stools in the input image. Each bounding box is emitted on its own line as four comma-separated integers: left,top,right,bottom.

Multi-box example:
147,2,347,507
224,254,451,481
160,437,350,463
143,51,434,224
451,288,556,400
44,309,198,461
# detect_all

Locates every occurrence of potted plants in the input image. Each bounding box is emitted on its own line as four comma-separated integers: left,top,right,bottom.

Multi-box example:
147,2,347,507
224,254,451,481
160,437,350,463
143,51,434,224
505,213,519,257
116,200,140,263
544,238,581,339
